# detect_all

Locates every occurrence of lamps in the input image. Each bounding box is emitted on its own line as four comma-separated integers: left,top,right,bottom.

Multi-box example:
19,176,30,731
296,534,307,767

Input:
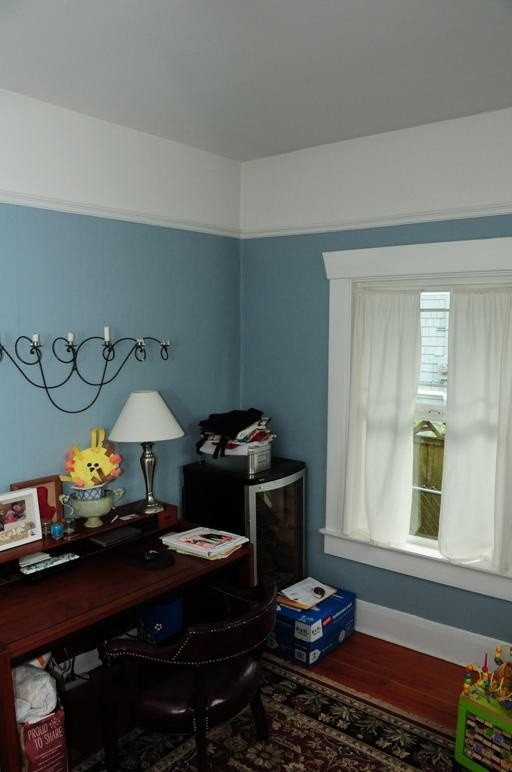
106,390,185,514
0,326,172,414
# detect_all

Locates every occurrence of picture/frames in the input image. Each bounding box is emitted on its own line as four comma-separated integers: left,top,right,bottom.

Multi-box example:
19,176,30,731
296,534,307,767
10,475,64,529
0,487,43,552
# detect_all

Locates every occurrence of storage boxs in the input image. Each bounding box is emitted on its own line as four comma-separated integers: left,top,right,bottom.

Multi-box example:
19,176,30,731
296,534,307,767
262,584,356,668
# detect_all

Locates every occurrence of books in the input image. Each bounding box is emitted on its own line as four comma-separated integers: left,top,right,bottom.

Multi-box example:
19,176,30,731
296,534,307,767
89,526,142,547
159,525,250,560
277,577,338,609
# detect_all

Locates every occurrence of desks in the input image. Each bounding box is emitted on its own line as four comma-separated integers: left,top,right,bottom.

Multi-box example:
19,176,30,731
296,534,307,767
0,499,256,772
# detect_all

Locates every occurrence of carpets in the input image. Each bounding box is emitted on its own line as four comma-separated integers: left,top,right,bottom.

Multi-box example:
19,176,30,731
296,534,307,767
62,650,471,772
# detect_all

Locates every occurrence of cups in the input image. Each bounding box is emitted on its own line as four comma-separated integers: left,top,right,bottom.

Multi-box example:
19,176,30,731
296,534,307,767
41,517,76,541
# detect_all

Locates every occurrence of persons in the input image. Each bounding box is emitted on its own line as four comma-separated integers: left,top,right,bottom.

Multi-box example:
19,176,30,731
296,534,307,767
3,500,25,525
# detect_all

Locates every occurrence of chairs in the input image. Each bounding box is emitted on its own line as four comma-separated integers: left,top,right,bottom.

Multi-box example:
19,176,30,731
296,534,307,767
89,577,278,772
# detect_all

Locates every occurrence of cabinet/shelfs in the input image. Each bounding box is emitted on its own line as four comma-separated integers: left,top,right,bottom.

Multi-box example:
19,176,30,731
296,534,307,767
179,456,307,592
